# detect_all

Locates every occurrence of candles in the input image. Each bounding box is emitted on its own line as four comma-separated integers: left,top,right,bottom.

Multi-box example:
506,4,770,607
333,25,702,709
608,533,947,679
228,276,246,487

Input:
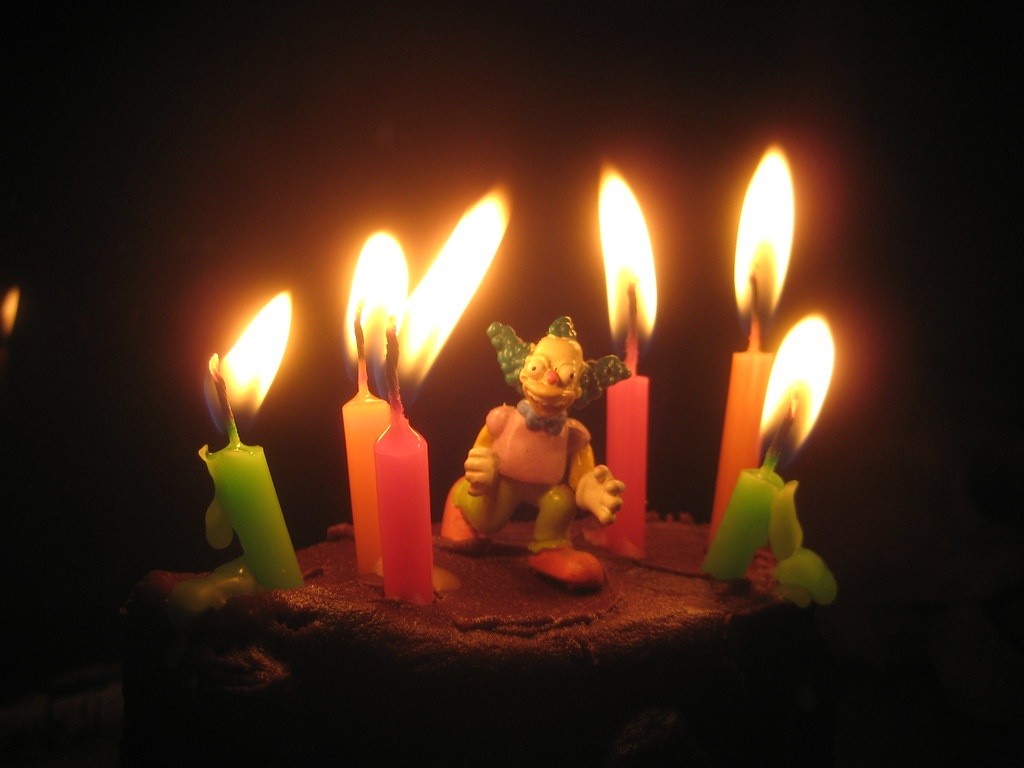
599,285,650,560
702,409,794,608
338,311,391,578
705,275,775,554
373,328,435,607
197,355,307,590
